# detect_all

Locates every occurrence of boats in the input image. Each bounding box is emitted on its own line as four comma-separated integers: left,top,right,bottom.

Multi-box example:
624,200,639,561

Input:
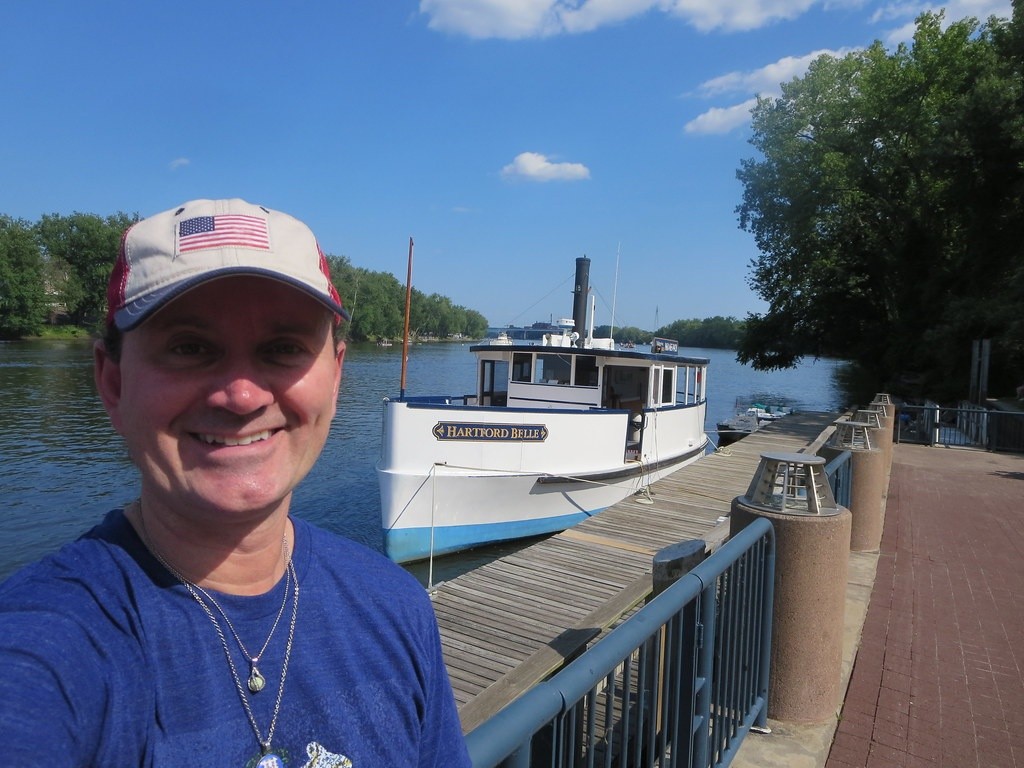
377,234,711,566
714,400,798,443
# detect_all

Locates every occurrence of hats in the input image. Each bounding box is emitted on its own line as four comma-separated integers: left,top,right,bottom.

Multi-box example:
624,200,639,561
107,197,350,331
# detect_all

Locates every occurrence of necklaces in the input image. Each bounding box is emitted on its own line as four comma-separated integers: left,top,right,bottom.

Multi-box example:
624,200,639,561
135,498,299,768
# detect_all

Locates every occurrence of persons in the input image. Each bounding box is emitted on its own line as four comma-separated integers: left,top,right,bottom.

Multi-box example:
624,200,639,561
0,198,482,768
